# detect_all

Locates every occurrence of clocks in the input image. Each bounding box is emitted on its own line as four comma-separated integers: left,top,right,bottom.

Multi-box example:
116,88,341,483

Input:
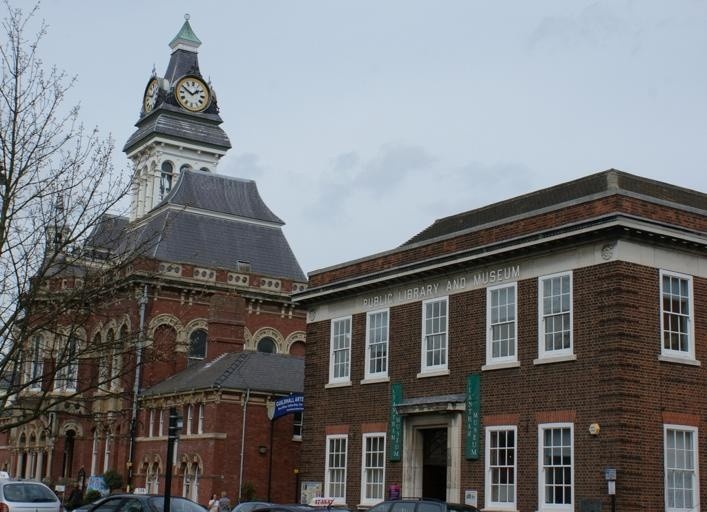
174,74,213,114
142,76,160,114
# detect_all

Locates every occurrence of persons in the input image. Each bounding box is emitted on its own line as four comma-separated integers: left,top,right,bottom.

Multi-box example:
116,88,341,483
66,484,83,512
206,490,232,512
